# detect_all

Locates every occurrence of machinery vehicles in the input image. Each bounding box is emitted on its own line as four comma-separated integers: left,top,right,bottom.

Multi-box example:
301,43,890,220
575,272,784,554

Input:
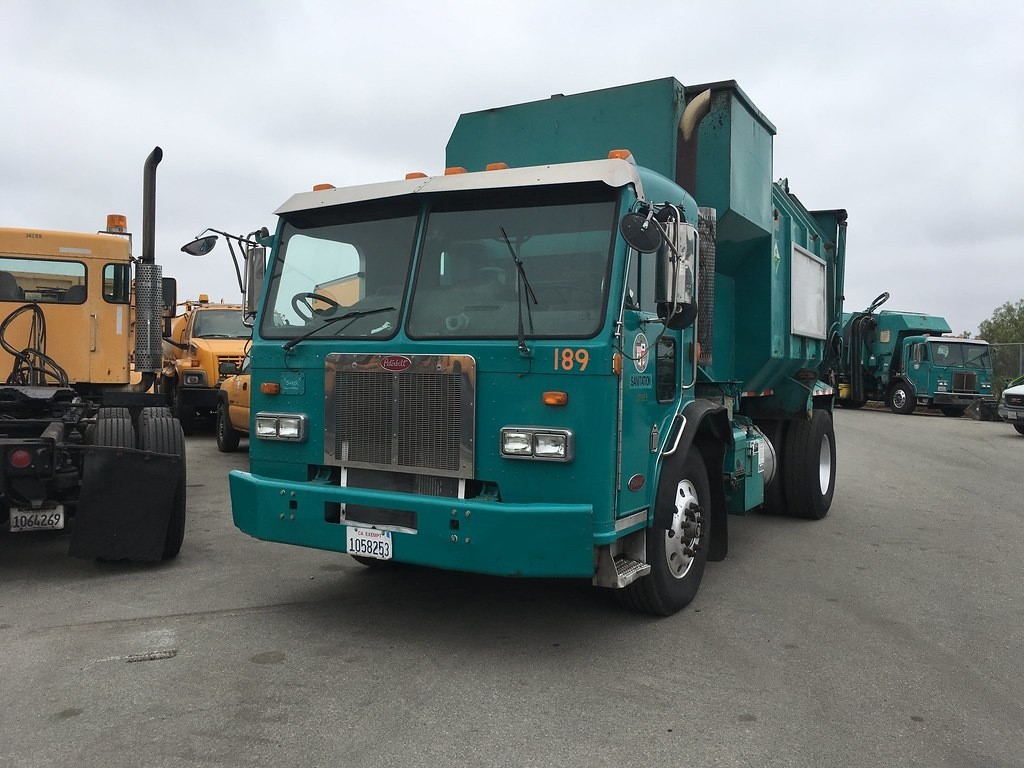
152,293,290,436
0,146,187,564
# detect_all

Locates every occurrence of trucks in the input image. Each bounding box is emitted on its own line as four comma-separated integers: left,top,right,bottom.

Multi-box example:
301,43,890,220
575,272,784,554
836,291,999,415
181,76,848,617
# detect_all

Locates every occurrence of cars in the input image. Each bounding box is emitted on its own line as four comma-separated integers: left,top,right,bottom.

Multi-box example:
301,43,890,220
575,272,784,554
999,385,1024,436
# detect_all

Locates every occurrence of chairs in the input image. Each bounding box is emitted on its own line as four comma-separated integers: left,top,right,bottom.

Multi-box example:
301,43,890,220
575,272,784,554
66,285,86,302
0,271,20,300
